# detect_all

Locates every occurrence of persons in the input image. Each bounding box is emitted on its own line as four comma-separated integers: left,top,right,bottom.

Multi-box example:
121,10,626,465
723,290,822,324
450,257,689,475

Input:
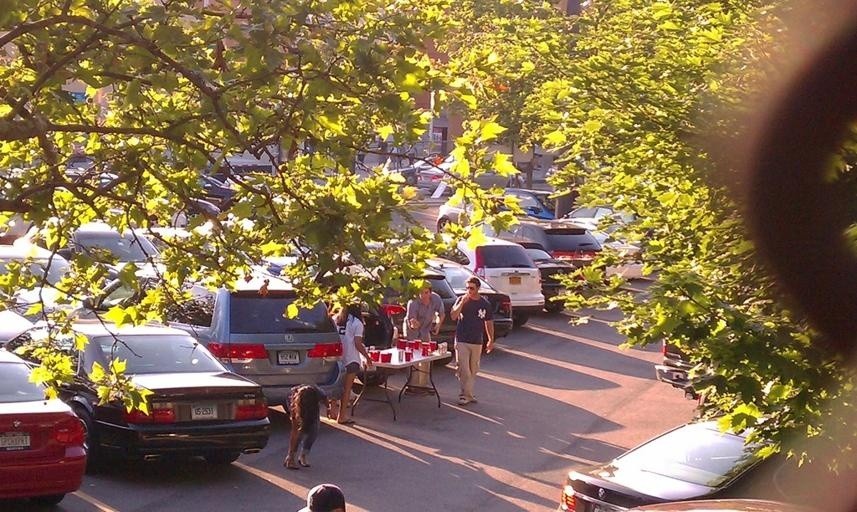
282,383,329,470
326,300,373,424
402,281,445,393
297,484,346,512
452,275,495,404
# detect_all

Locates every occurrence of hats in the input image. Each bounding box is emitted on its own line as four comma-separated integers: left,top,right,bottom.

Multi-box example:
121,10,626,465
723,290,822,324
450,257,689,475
296,482,347,511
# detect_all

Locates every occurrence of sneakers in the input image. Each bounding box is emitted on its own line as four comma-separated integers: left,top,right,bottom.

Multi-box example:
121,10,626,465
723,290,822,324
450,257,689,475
456,394,478,406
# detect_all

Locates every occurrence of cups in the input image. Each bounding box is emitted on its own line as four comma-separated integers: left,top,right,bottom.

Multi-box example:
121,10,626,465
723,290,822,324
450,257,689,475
363,339,450,362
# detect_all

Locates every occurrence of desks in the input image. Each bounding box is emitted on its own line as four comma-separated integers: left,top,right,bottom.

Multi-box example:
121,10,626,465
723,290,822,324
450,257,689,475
351,346,452,422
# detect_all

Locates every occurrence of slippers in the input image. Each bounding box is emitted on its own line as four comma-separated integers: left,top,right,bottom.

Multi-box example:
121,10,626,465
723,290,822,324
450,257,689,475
337,417,355,425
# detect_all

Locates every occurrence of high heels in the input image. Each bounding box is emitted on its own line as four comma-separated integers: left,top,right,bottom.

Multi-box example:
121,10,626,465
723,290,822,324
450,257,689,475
283,455,311,470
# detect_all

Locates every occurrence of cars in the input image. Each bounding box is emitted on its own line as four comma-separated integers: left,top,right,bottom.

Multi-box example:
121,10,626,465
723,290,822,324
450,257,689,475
263,147,648,387
627,499,807,511
656,334,718,404
2,145,342,507
560,413,836,511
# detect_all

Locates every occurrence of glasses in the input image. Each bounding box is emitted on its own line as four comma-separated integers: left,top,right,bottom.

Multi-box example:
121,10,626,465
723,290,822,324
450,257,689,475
466,286,475,290
